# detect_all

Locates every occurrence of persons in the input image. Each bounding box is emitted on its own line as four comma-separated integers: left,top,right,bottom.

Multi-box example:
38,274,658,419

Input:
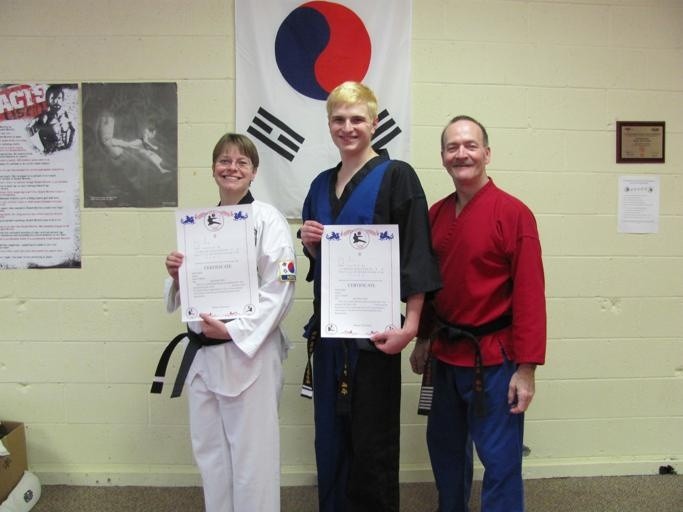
112,118,172,175
27,86,77,152
296,81,443,512
409,116,546,512
164,133,296,512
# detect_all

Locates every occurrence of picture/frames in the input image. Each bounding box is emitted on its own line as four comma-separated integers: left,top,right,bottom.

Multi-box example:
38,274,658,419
616,120,666,164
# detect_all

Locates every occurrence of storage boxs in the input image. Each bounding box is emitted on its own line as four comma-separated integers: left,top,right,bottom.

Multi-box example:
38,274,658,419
0,420,29,508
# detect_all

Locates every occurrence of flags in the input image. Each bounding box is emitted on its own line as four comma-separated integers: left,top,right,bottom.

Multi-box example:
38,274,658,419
235,0,412,219
